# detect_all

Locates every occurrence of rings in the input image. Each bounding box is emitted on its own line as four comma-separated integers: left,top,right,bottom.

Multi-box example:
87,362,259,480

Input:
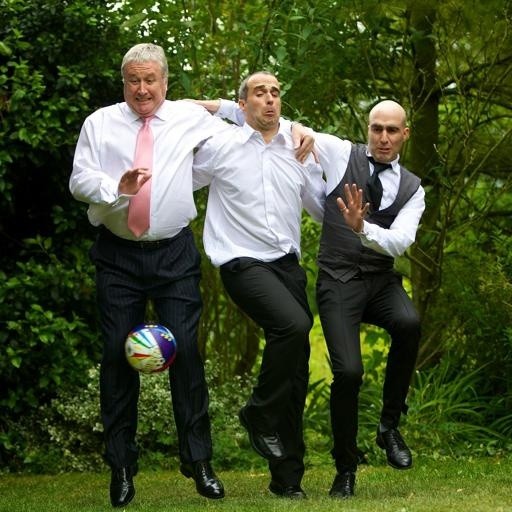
340,205,347,211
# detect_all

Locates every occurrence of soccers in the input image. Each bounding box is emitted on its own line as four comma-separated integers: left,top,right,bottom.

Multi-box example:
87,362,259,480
126,323,176,373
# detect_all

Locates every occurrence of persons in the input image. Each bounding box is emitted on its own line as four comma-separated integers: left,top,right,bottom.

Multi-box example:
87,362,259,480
68,43,322,509
193,70,330,499
181,96,430,497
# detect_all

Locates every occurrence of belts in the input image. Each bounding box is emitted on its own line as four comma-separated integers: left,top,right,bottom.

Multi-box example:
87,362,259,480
141,240,170,250
352,274,364,280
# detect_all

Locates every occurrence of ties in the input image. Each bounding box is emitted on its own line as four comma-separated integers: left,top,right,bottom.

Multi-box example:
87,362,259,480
366,158,392,213
127,116,154,239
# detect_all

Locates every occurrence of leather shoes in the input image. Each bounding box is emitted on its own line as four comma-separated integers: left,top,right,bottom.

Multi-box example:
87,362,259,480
268,479,307,499
376,426,412,470
179,460,225,498
248,428,286,460
329,472,356,500
110,461,138,508
